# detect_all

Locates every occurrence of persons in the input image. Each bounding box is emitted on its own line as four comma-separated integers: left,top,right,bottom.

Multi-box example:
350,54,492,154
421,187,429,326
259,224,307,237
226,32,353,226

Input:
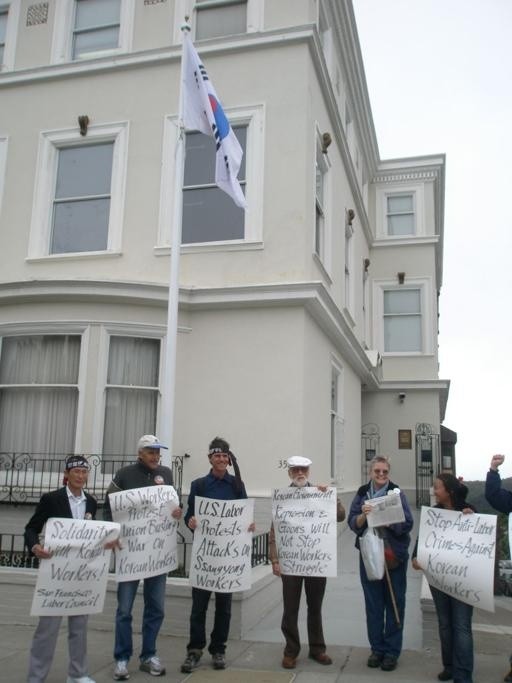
484,453,511,683
410,470,481,681
179,436,258,673
25,456,120,683
347,455,415,671
269,455,346,670
100,433,183,681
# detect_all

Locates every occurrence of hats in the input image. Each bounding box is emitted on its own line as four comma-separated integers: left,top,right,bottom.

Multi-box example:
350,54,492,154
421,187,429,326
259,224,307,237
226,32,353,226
288,456,313,468
137,434,169,449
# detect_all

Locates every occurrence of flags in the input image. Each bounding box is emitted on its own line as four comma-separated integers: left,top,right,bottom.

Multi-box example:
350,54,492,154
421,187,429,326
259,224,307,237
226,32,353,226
178,27,247,210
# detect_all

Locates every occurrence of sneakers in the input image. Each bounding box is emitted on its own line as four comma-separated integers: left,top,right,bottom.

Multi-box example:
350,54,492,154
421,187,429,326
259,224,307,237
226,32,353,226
141,656,165,676
283,655,296,668
438,670,454,681
181,654,200,673
313,654,330,664
114,659,129,679
67,675,96,683
212,652,226,668
367,653,398,670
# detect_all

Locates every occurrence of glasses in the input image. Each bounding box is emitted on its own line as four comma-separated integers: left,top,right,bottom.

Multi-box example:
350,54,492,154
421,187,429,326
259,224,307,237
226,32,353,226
373,468,389,474
292,467,309,473
